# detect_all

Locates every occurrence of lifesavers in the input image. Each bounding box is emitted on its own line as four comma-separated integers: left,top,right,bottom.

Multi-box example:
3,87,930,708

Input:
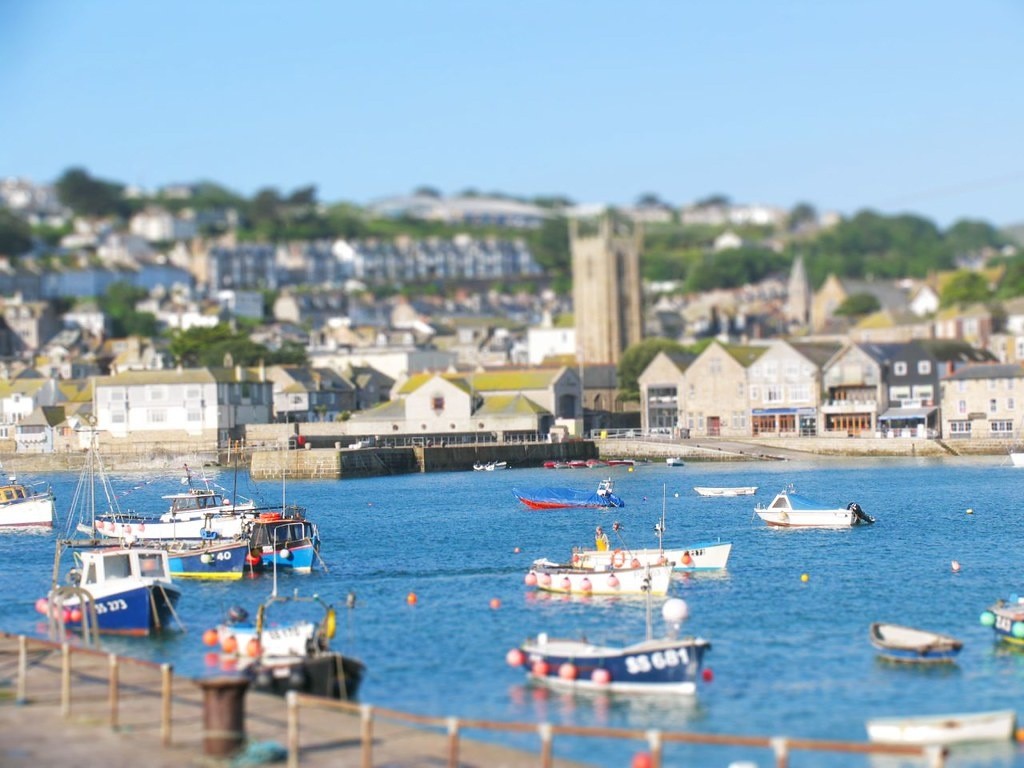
259,513,280,521
611,549,625,568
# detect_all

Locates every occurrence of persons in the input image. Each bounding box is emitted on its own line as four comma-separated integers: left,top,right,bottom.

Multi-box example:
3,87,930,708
594,526,610,551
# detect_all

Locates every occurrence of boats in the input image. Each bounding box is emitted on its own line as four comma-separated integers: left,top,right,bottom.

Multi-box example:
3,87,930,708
861,706,1024,757
504,565,717,698
691,486,761,498
544,457,655,469
512,476,625,509
751,490,876,530
867,621,963,666
666,456,688,469
1005,448,1023,468
980,592,1023,653
0,462,57,531
204,521,368,698
40,420,325,644
530,480,734,599
473,458,509,475
